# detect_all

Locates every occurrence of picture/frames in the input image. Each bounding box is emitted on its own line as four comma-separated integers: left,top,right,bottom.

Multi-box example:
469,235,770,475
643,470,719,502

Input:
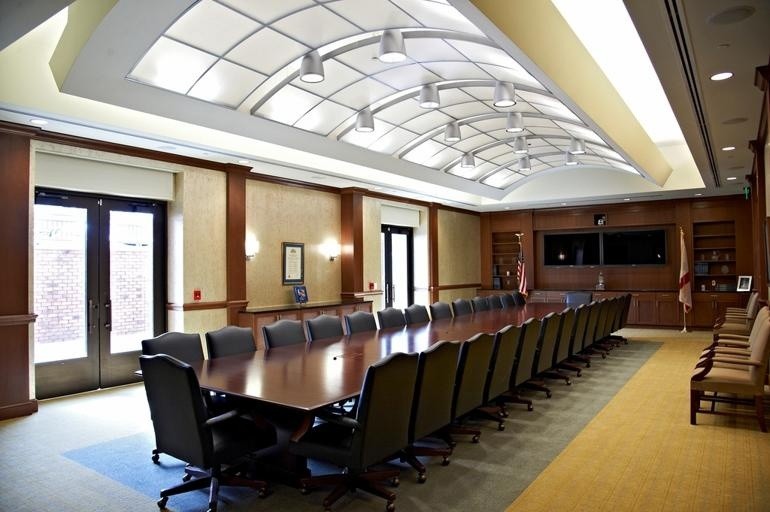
294,285,307,302
493,276,501,290
281,240,305,285
736,274,752,292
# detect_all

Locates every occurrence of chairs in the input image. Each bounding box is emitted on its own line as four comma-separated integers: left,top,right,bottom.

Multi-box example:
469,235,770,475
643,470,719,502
410,341,463,487
306,313,345,340
205,326,258,359
377,292,525,329
135,348,280,511
454,328,498,445
345,310,377,335
261,318,307,349
687,295,770,434
138,331,265,470
291,346,421,512
485,294,631,419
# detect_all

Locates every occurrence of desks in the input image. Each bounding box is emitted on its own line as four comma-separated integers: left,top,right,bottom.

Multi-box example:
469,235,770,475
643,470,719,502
131,300,583,511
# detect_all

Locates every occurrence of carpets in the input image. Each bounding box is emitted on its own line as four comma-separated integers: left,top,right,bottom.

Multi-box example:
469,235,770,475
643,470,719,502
58,335,666,510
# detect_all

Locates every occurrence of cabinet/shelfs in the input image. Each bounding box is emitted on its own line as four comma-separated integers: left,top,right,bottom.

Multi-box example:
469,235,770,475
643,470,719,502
694,221,738,292
627,293,638,325
657,291,679,328
691,292,743,329
491,232,522,292
637,291,657,328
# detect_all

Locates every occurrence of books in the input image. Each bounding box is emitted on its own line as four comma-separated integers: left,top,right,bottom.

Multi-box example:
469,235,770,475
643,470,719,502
714,282,737,291
694,262,709,274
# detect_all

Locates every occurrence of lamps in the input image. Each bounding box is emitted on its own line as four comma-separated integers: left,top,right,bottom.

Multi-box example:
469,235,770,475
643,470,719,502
451,137,600,169
296,23,409,84
510,154,590,182
327,238,341,262
351,77,524,132
244,233,260,261
440,113,532,144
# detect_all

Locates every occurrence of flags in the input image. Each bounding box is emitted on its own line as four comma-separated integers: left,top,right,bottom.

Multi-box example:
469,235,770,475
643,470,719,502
678,224,693,314
516,243,529,298
